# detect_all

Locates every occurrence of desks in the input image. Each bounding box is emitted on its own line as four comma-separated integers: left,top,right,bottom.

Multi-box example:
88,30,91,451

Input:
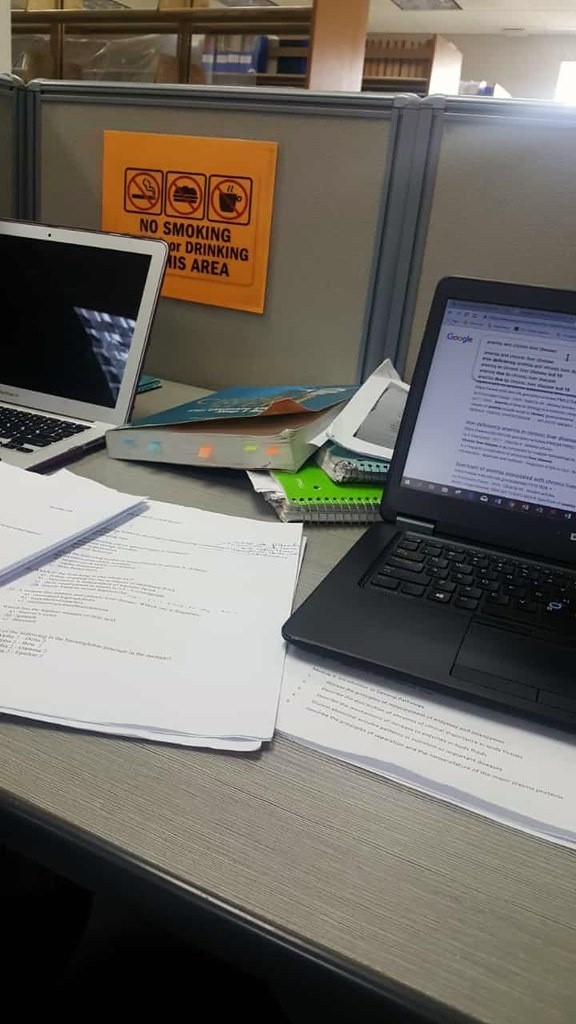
1,377,576,1023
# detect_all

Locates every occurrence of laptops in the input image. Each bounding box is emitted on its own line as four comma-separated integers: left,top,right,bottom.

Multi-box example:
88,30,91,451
282,274,576,736
0,216,169,473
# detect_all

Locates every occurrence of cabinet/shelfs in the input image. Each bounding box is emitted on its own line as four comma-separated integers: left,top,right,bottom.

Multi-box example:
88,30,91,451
199,31,463,96
0,0,371,95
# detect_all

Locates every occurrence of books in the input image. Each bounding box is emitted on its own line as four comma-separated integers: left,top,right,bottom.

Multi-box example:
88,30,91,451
106,356,407,524
